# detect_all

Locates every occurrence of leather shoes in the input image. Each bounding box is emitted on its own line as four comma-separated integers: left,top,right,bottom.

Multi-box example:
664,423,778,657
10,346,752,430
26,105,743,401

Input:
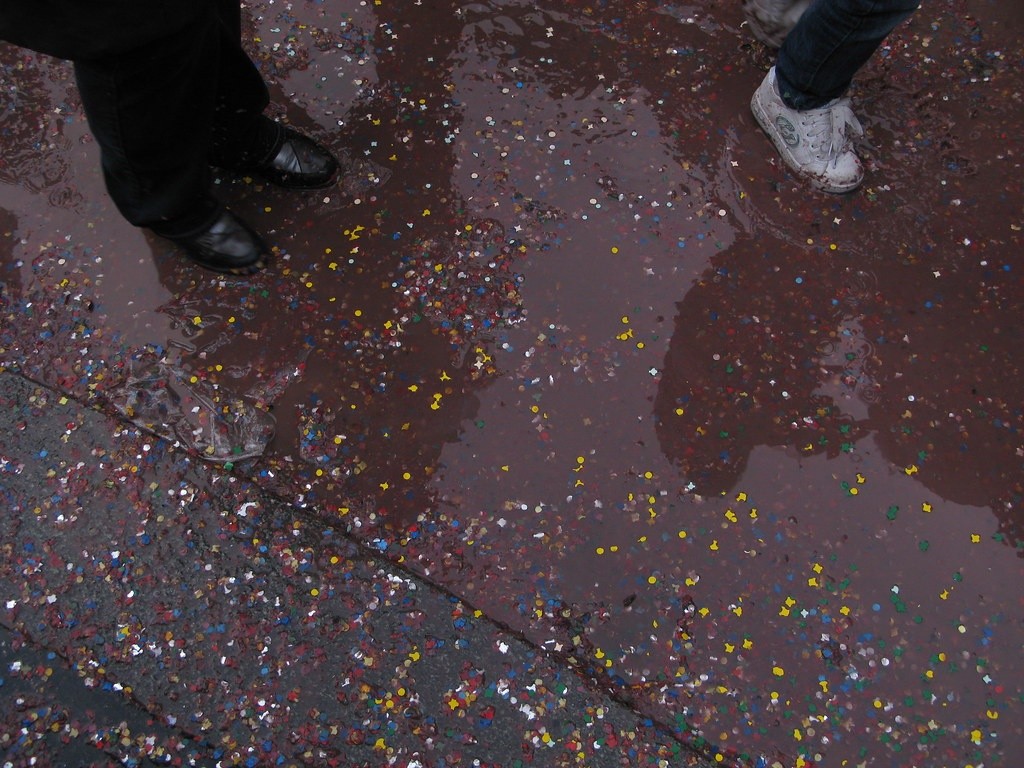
256,126,341,189
149,205,266,275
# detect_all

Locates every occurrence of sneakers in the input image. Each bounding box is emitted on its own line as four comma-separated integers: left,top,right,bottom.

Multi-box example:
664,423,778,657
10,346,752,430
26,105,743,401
750,65,865,194
741,0,815,48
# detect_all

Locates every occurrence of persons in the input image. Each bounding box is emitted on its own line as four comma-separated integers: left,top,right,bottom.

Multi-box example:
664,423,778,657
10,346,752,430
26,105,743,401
0,0,339,277
744,0,921,193
